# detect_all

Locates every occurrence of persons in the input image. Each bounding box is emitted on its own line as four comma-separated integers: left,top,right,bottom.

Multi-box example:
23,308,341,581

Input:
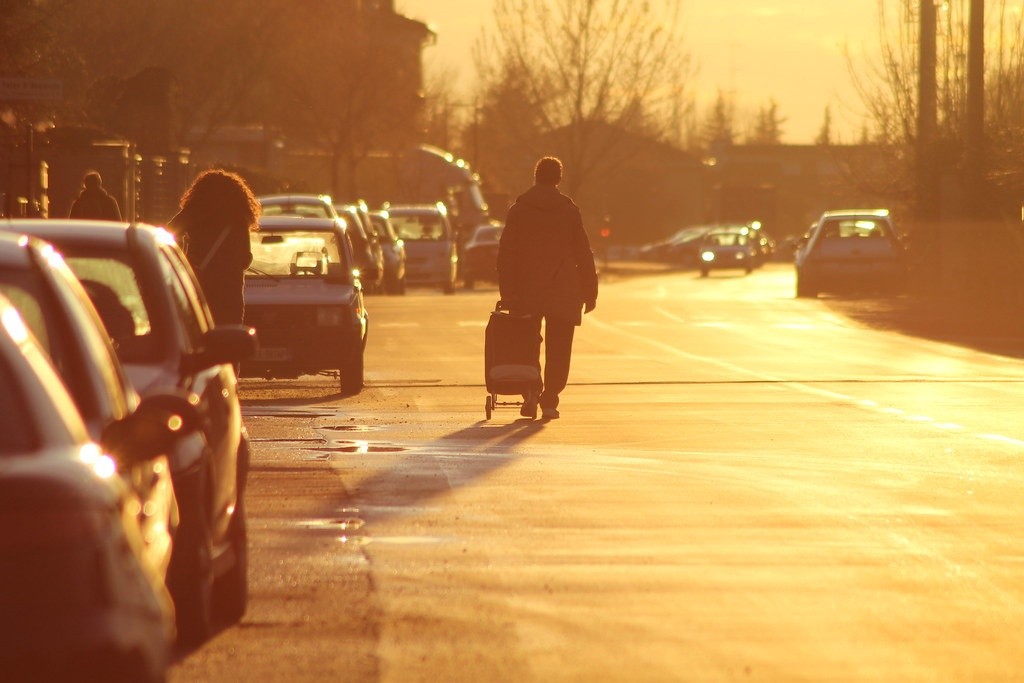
497,156,599,420
66,156,658,420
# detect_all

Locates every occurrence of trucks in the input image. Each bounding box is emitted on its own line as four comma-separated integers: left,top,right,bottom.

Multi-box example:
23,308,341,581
274,142,491,235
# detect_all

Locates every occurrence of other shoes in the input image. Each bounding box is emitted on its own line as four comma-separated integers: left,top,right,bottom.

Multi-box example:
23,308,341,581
542,407,559,420
520,395,540,417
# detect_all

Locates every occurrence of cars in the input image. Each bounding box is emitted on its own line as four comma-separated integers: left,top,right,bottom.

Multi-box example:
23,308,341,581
237,192,458,396
0,218,252,683
794,207,904,299
635,221,772,277
458,224,505,291
